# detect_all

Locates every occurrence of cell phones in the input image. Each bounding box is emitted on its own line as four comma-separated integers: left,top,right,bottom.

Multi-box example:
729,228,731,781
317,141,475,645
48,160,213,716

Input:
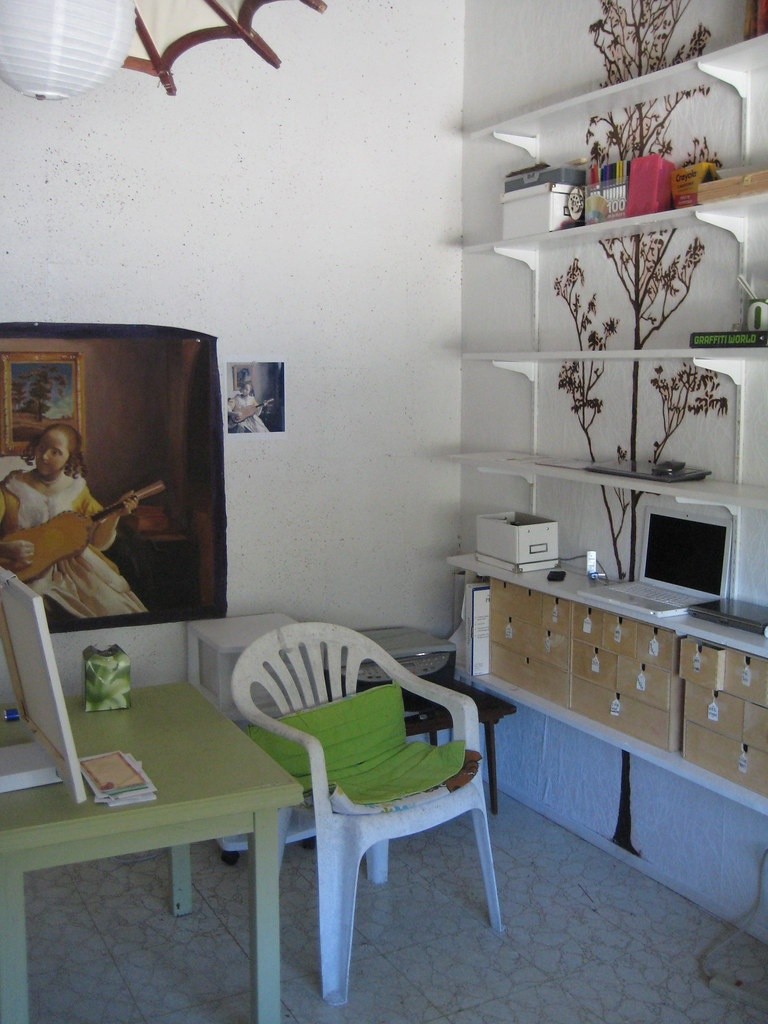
547,571,566,581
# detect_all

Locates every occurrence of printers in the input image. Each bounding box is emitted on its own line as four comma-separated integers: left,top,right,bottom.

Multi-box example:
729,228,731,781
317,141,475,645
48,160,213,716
324,626,458,711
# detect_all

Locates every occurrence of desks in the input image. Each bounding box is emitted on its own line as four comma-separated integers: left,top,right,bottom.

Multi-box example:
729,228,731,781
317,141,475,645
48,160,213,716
405,680,517,814
0,680,304,1024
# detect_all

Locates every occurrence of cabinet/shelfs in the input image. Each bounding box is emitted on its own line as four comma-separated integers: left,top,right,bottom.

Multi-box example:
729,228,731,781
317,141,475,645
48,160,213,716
188,613,318,866
447,33,768,946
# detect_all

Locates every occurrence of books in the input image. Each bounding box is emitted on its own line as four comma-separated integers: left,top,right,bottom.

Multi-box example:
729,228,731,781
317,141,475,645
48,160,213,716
589,160,630,223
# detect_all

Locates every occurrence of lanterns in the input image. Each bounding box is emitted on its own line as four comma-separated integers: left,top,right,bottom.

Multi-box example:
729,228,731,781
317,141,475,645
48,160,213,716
0,0,138,101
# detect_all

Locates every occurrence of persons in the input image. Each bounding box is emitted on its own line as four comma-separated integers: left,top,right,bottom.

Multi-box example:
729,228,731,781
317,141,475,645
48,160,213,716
226,383,270,433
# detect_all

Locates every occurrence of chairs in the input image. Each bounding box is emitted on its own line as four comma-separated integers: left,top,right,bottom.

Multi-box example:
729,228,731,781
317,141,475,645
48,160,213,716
231,622,505,1005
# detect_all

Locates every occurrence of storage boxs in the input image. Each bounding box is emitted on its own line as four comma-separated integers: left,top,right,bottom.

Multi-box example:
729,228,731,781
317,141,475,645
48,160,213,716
500,166,585,240
697,170,768,204
465,576,768,798
625,154,675,217
475,511,558,574
672,162,716,208
585,176,629,225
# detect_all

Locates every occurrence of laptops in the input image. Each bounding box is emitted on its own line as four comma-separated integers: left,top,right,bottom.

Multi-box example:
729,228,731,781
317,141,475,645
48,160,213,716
687,597,768,635
578,506,732,619
